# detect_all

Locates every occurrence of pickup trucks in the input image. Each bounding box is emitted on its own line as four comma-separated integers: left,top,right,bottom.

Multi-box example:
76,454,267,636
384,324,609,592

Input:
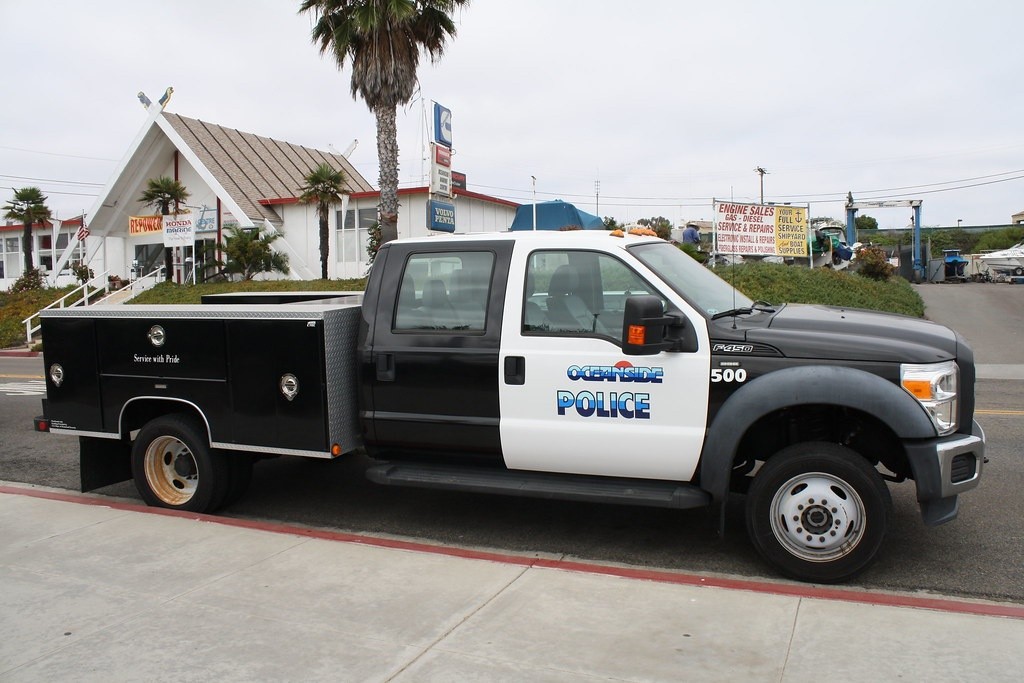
29,226,989,587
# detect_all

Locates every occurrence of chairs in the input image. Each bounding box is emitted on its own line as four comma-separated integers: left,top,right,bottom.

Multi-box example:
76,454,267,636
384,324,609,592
397,274,429,326
449,269,484,328
546,264,608,335
422,278,463,325
525,272,555,328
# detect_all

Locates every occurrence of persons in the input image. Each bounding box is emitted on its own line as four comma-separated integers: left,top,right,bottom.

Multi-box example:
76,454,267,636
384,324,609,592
682,222,702,247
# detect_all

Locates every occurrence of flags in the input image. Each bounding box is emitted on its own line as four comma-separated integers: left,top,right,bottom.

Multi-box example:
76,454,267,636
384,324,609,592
77,221,90,241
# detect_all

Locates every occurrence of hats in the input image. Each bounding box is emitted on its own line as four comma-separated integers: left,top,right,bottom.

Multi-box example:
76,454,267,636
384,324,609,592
688,222,700,228
